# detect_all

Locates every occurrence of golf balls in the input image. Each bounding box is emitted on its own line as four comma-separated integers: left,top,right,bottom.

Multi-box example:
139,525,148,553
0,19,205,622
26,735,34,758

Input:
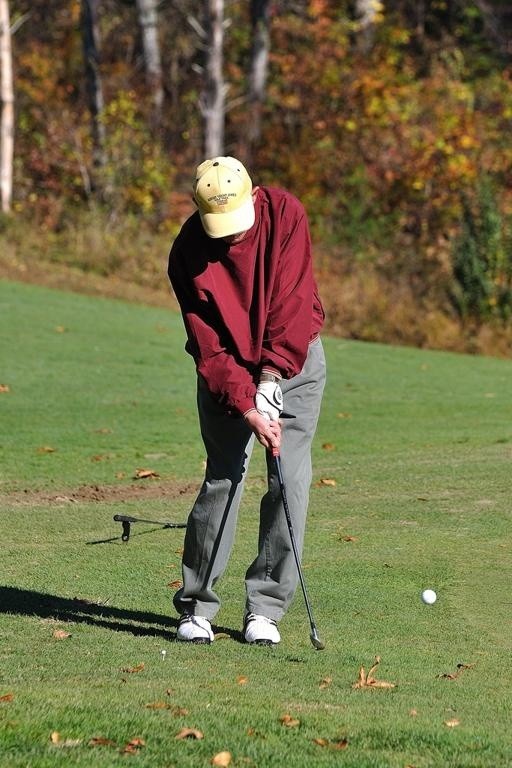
421,589,438,604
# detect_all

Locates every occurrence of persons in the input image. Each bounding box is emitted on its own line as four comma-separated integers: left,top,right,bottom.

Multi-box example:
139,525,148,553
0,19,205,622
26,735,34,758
168,156,327,645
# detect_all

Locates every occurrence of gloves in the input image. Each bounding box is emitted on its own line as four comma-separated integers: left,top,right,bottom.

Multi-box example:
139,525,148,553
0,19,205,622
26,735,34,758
255,380,284,424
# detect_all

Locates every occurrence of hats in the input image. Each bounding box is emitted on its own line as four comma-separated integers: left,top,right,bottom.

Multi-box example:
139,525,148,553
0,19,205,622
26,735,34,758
192,155,256,239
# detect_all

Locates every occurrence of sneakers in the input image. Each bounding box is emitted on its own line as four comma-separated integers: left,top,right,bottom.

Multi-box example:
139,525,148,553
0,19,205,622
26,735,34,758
242,607,281,646
176,613,215,645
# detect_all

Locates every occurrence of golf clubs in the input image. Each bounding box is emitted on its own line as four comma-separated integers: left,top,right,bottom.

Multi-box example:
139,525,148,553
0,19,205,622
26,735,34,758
272,446,323,648
